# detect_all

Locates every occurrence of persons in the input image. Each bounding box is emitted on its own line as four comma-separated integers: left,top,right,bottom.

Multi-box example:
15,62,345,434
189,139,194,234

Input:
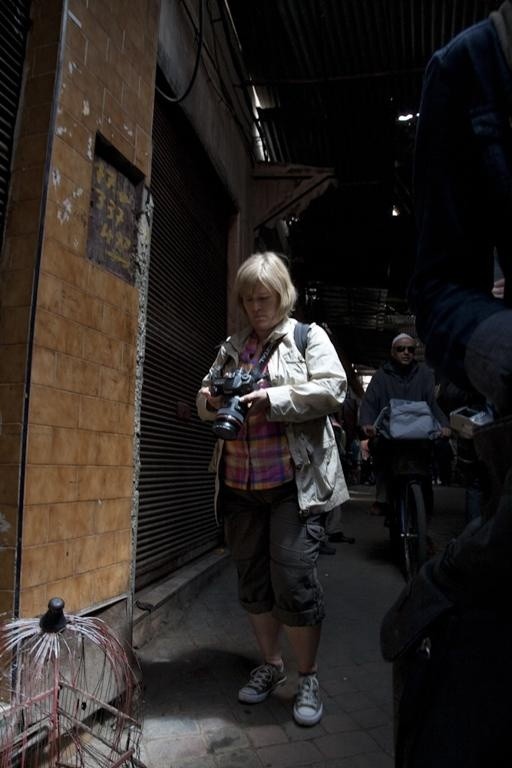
381,471,511,768
319,333,452,553
405,1,510,402
196,252,348,726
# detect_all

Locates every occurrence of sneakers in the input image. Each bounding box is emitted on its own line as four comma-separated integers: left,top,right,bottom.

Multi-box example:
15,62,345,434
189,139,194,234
239,661,289,703
320,541,336,554
329,532,349,543
294,664,325,725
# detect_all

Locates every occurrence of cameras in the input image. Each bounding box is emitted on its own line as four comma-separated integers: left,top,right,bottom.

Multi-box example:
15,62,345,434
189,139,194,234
210,366,258,441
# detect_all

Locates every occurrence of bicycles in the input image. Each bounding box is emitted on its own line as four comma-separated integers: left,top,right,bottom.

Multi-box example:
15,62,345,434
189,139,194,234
358,426,453,583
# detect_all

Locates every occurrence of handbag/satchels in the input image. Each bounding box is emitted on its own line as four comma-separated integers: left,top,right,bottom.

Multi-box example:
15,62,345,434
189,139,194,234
390,399,434,436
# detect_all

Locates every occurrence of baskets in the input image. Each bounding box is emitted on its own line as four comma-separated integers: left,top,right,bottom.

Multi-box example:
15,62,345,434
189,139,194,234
389,441,434,476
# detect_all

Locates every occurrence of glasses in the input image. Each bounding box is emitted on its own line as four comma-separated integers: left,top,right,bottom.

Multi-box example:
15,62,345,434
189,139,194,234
396,346,417,353
